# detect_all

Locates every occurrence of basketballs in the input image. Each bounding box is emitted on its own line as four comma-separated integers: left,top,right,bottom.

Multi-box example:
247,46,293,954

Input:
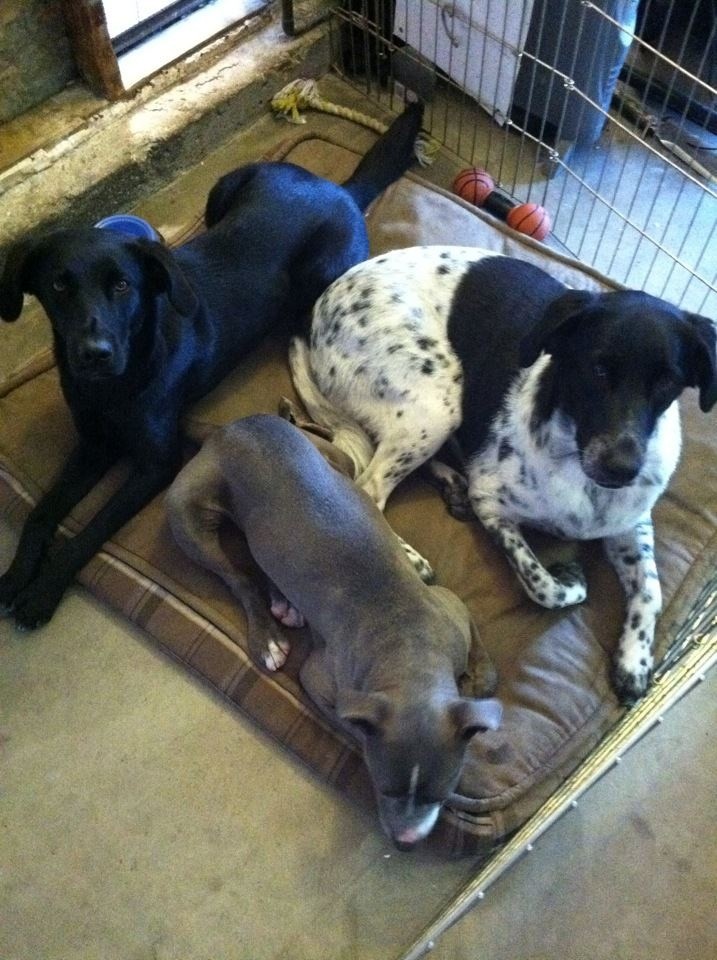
506,203,550,242
453,167,494,207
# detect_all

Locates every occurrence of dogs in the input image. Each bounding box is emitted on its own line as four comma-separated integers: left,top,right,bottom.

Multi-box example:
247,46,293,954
166,416,504,851
0,103,429,631
279,243,717,711
